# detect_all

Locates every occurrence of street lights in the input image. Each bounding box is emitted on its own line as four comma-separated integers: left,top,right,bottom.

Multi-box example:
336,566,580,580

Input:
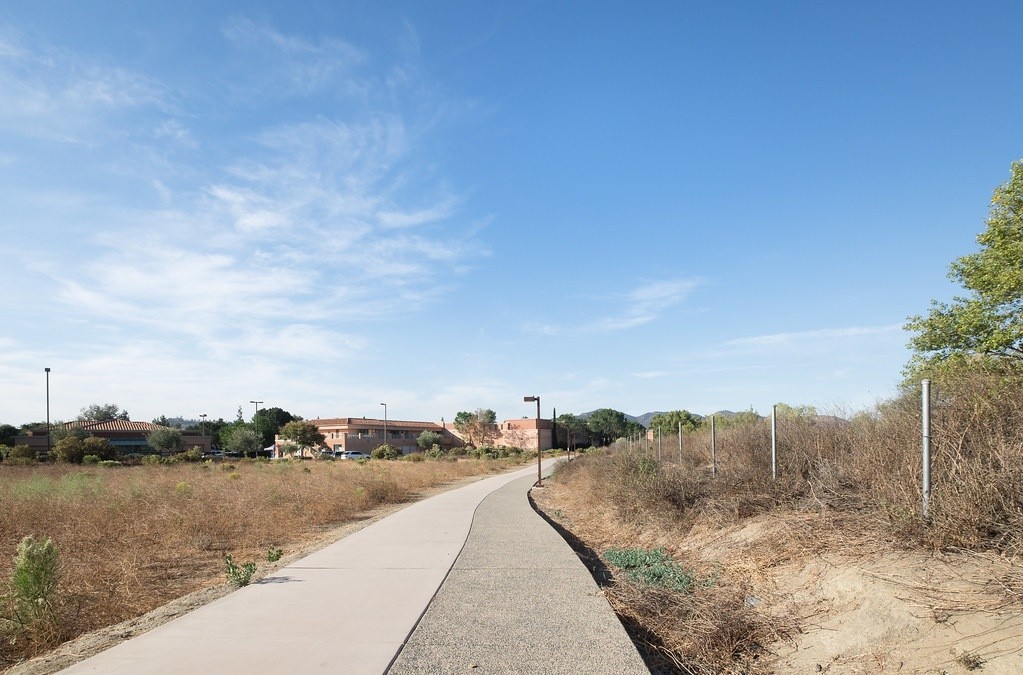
250,400,264,457
45,366,53,450
199,413,207,436
522,396,545,488
380,402,387,446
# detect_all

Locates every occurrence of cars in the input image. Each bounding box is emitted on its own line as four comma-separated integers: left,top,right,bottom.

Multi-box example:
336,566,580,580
320,450,371,461
201,450,273,460
